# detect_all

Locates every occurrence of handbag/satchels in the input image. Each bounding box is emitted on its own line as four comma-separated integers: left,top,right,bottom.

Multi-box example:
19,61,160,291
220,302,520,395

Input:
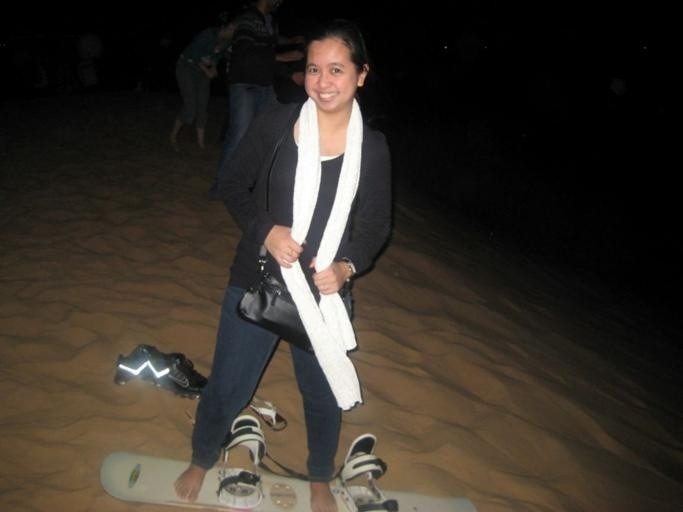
237,273,313,353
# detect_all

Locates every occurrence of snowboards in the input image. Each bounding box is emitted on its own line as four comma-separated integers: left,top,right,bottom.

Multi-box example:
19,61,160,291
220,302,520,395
100,415,477,511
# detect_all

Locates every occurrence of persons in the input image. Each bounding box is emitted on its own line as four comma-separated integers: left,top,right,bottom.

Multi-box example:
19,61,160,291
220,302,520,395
170,20,391,512
209,0,305,200
169,11,238,151
220,12,306,141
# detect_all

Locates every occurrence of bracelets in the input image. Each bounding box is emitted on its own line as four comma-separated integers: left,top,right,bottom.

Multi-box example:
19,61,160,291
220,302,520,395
344,259,353,280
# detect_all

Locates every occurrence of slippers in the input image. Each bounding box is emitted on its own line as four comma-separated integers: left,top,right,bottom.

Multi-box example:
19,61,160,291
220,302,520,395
249,396,287,430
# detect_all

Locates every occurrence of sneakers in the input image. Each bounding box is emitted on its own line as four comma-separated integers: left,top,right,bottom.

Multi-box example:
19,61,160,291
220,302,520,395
115,345,208,400
